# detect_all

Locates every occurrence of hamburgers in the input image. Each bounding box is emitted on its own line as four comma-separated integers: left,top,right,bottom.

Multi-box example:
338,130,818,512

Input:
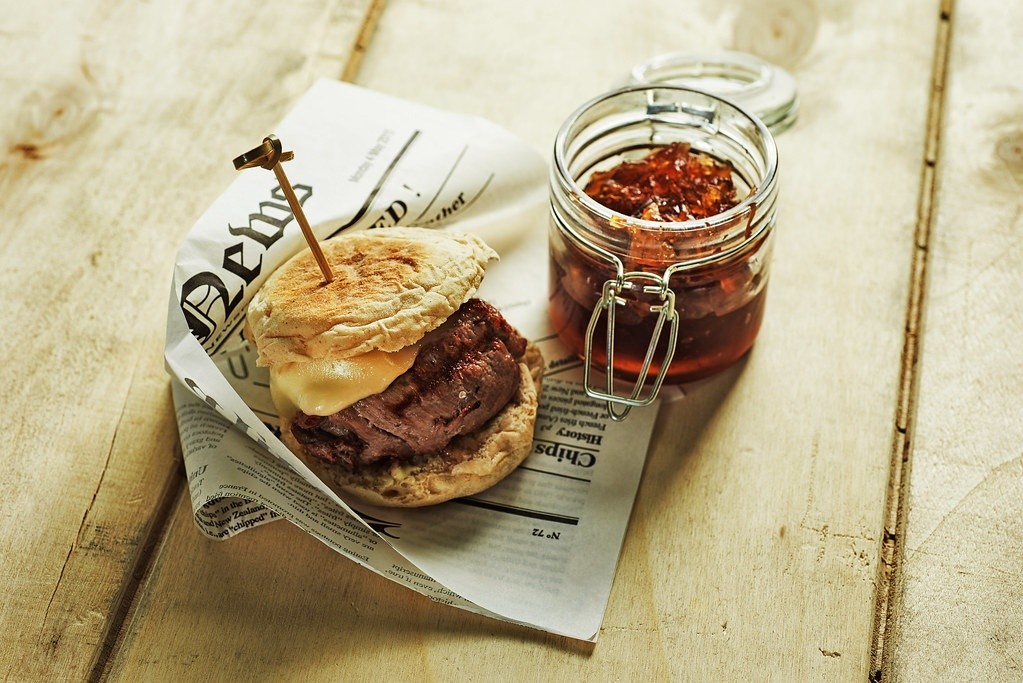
244,224,547,508
